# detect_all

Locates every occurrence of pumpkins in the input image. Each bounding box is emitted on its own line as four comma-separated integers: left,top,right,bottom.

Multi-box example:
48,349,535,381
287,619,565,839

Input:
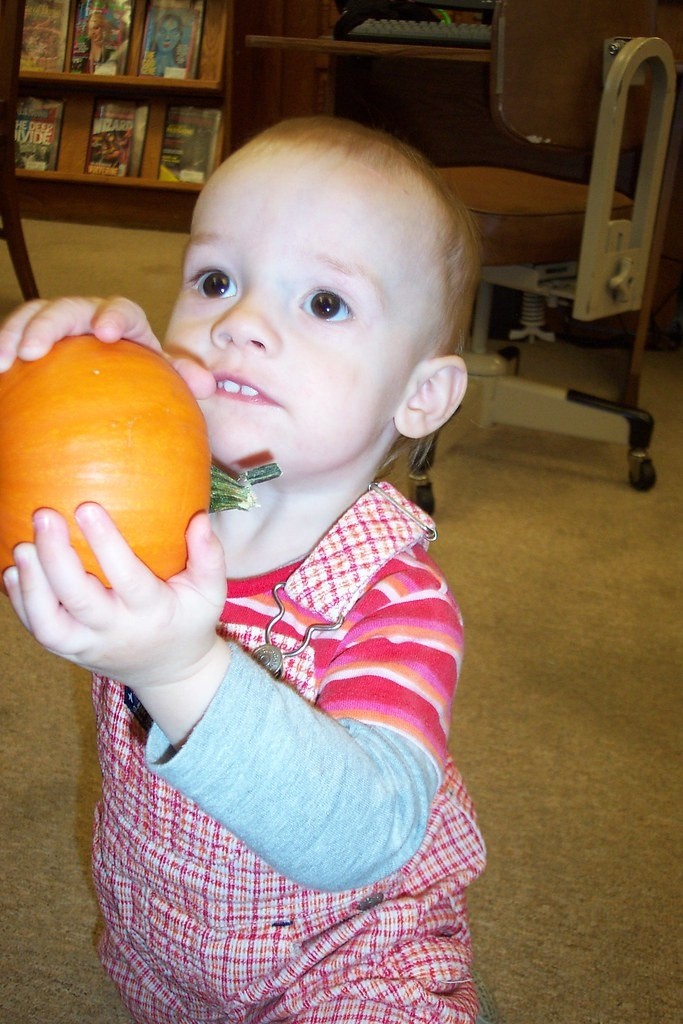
0,335,259,594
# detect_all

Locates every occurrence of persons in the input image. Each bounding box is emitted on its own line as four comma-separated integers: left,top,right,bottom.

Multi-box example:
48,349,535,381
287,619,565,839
0,116,487,1024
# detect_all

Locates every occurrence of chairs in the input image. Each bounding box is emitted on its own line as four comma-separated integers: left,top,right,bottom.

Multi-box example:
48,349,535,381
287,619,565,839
430,36,674,488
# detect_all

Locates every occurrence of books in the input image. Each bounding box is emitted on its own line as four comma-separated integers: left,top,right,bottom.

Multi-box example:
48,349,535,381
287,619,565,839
20,0,204,79
14,96,222,182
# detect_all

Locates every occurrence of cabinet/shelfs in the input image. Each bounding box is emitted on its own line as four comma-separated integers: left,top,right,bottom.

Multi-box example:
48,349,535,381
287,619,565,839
11,0,247,190
